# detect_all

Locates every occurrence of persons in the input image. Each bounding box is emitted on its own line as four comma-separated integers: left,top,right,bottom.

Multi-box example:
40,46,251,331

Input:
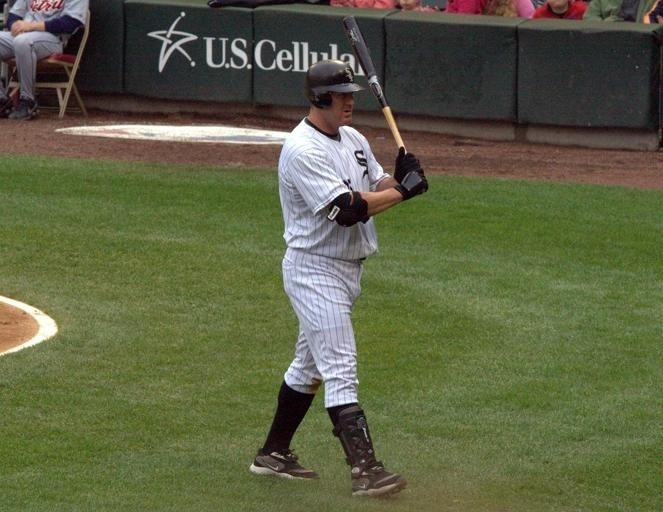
0,0,88,120
248,58,428,497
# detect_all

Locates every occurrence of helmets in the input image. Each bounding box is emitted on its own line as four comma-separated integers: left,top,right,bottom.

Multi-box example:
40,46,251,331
301,60,365,109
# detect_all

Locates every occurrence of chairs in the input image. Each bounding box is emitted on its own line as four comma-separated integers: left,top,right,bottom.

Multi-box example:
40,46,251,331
0,9,91,121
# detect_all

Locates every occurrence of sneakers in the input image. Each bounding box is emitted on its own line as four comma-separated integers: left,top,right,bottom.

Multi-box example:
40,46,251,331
248,448,319,481
346,466,408,498
0,97,40,120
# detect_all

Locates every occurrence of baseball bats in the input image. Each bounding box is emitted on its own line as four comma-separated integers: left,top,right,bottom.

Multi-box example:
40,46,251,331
341,13,406,154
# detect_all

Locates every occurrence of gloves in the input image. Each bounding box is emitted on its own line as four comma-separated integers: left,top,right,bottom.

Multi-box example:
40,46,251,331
395,169,429,200
392,145,420,183
